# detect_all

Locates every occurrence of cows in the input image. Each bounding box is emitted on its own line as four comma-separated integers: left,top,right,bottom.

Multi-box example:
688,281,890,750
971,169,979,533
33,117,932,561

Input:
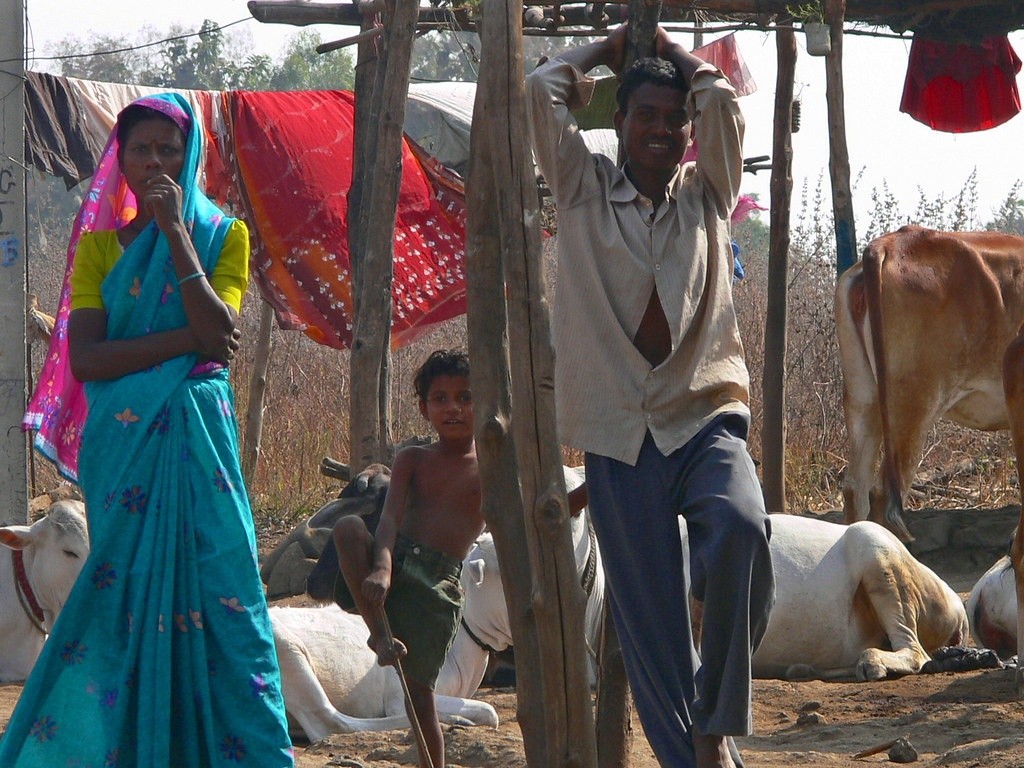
247,460,970,742
0,498,91,693
834,226,1023,705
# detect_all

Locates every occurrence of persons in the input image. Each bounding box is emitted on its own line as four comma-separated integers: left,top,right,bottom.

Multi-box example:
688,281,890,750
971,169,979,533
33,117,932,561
0,92,293,768
525,19,777,768
306,349,590,768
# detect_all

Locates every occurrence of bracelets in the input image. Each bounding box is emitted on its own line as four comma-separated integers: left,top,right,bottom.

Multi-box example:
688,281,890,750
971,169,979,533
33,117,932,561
176,273,207,288
